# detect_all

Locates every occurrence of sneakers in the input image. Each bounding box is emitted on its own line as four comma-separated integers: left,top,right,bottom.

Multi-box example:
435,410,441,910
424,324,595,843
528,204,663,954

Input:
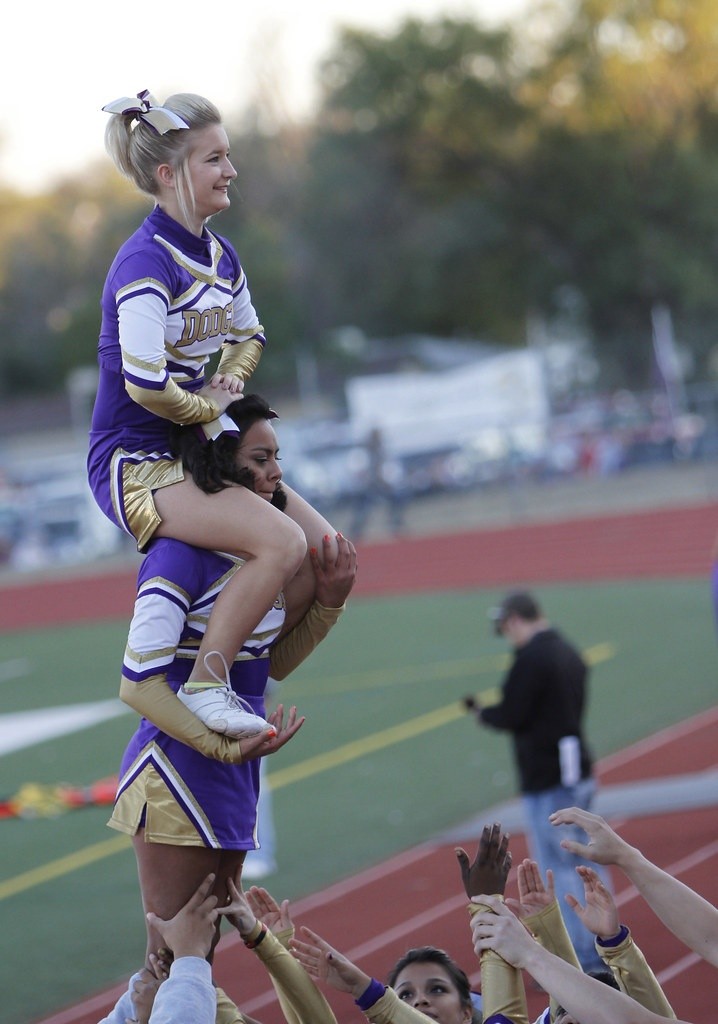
176,651,277,740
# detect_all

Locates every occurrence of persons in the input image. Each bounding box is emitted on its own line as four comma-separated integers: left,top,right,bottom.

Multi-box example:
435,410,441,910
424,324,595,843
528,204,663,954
105,391,288,980
96,806,718,1024
85,90,354,742
461,593,623,993
2,379,717,571
